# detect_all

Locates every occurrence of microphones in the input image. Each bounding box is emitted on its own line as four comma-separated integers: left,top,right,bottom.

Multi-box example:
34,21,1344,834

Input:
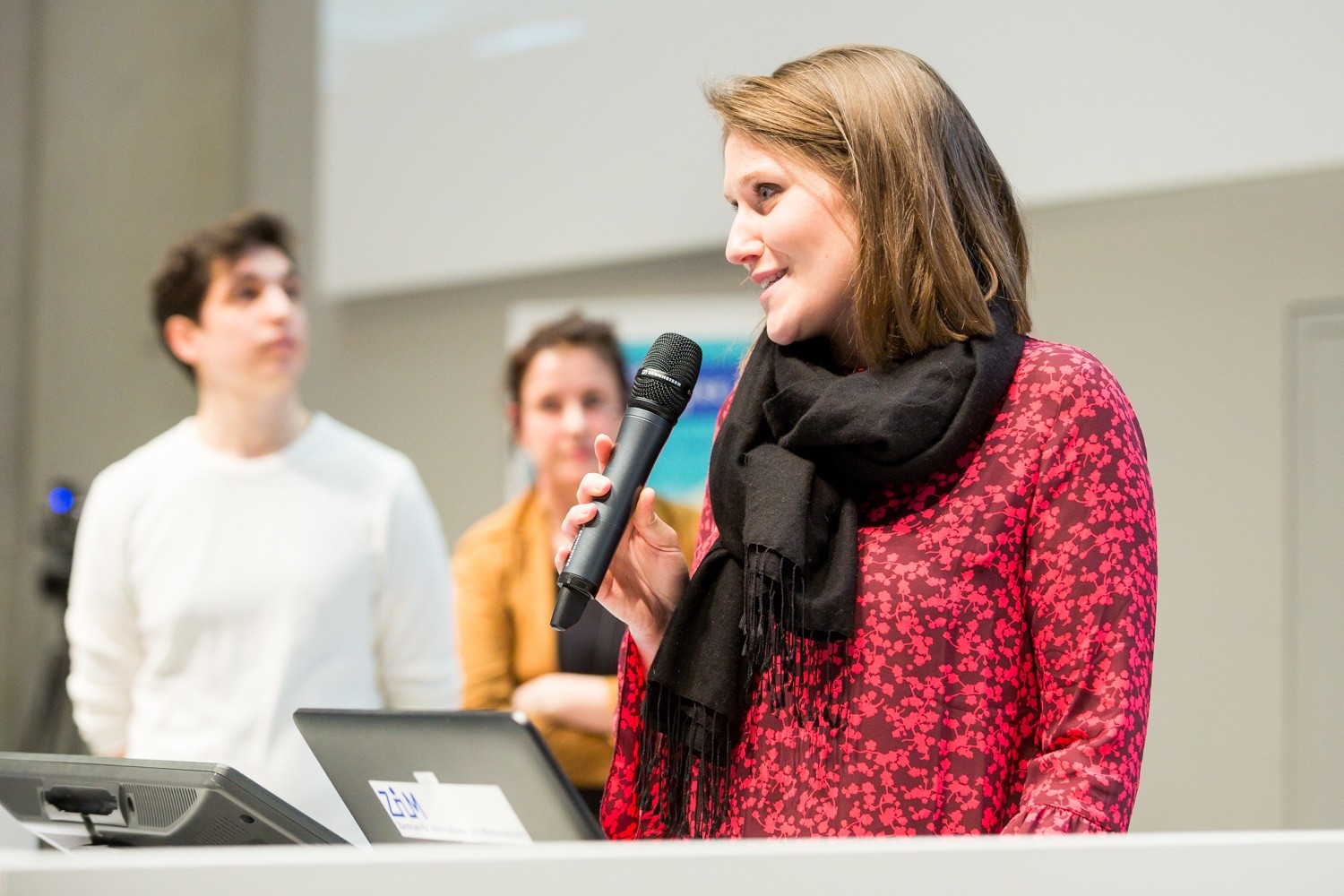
549,332,703,631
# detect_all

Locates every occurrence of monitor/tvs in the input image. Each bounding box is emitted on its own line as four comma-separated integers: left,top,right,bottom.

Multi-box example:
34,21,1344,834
0,753,356,852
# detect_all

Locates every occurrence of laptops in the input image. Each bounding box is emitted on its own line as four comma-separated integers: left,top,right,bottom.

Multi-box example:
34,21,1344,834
292,706,605,847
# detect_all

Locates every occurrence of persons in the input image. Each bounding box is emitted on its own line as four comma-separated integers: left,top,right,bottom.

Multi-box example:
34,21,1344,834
65,205,463,850
553,46,1160,840
455,307,702,827
